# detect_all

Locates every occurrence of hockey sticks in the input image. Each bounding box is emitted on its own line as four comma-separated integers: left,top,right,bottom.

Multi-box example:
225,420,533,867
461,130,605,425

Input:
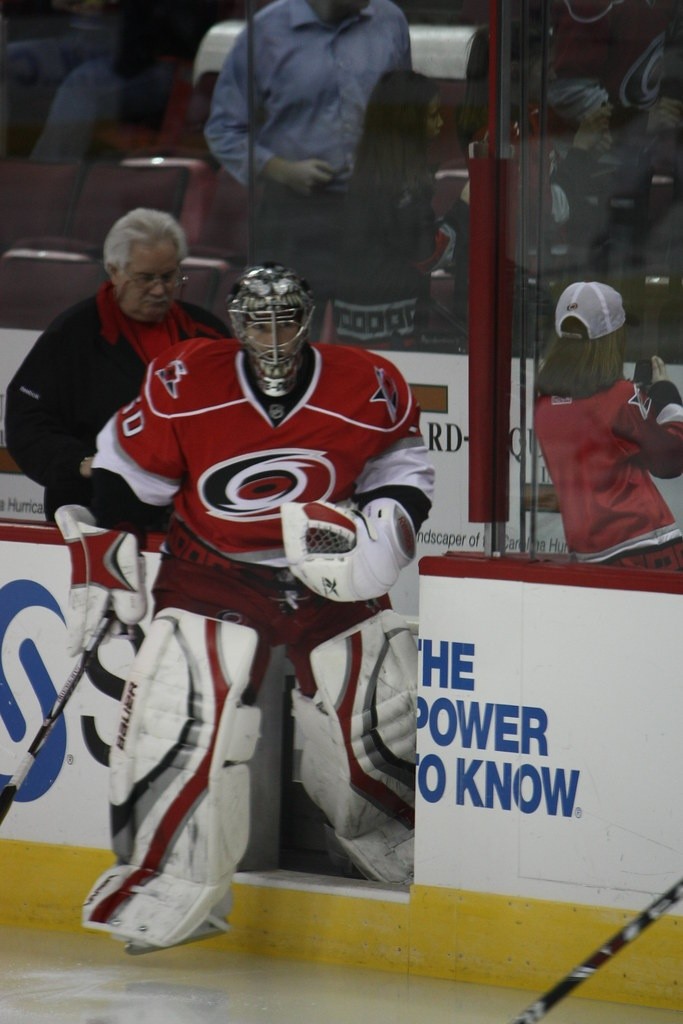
0,606,115,823
508,875,683,1024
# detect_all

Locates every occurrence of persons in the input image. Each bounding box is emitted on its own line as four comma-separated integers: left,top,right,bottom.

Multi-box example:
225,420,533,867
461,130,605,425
0,1,683,575
49,261,438,956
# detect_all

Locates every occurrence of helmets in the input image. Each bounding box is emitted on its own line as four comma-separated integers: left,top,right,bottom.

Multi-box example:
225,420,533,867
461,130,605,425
227,264,315,397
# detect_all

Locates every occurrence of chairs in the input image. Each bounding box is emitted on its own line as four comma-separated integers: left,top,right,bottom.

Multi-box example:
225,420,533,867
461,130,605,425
0,0,683,362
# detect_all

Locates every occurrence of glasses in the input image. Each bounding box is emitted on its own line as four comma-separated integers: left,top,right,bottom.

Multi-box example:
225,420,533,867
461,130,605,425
119,266,185,290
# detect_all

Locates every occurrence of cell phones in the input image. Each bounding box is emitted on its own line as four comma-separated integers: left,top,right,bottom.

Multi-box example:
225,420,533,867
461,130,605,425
633,358,654,390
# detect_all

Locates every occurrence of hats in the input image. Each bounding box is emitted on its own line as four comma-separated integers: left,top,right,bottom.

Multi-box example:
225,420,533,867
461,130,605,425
554,281,625,340
